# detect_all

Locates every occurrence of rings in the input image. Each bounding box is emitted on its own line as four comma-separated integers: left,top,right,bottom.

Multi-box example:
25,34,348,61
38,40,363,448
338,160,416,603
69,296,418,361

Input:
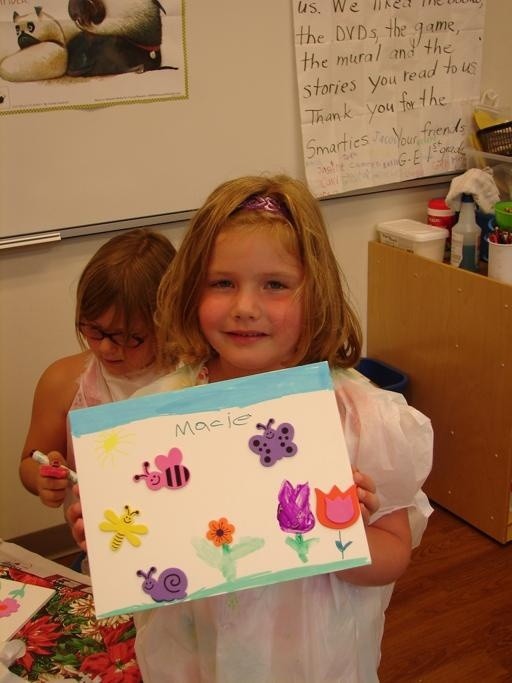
30,450,77,484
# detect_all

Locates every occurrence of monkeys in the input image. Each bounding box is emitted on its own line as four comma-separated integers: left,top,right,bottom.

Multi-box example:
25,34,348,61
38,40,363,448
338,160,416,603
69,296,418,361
39,464,70,479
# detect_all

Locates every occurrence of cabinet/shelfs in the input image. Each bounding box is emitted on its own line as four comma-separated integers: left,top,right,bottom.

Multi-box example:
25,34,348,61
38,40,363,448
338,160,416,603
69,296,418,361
427,198,457,264
449,193,482,274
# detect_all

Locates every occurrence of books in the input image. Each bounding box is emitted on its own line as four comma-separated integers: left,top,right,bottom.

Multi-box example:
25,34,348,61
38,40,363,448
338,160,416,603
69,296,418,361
463,150,512,202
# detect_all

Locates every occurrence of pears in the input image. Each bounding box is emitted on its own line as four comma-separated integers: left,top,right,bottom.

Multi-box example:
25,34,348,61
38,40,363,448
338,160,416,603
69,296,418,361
450,193,482,273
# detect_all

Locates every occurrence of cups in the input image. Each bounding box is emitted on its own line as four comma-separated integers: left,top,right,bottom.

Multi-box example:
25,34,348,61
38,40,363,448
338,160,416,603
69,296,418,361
74,319,147,348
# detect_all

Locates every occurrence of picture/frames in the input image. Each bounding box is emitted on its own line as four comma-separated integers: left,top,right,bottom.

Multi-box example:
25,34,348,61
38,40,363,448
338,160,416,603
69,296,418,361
366,240,512,544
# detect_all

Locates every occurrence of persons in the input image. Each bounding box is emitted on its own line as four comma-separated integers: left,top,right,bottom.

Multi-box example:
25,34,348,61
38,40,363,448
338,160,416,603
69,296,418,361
61,172,437,683
16,225,204,521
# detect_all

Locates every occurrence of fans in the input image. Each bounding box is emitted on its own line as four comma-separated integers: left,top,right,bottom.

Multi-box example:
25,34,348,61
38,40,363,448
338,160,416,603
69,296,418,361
0,0,512,250
